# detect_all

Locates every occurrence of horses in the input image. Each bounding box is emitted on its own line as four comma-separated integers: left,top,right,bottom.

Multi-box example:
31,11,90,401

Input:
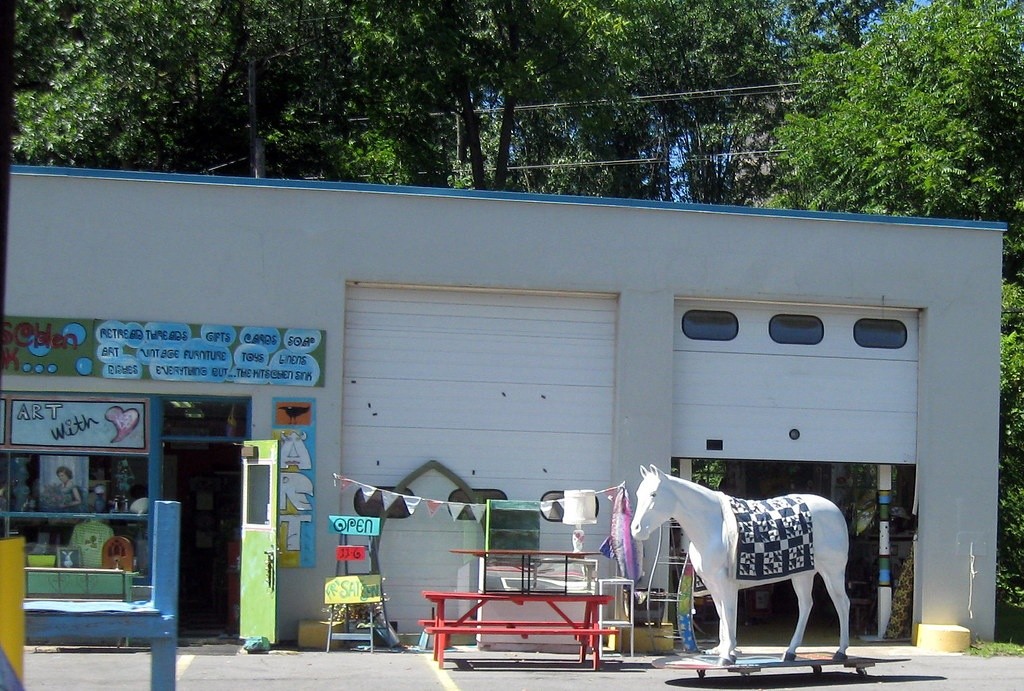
631,464,850,666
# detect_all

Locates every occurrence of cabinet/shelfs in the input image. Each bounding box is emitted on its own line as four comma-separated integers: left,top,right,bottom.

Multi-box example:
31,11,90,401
597,575,635,659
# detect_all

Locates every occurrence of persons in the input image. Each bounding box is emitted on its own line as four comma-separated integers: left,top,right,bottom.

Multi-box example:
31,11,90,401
56,466,81,513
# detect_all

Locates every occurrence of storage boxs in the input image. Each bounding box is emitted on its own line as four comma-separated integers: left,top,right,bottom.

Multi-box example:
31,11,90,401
297,619,344,651
608,622,673,652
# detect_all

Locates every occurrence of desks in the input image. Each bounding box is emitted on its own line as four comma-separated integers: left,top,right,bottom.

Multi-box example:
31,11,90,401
422,590,615,672
448,550,602,596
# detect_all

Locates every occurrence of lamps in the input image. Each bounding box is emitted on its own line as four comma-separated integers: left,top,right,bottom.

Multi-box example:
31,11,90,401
562,489,597,553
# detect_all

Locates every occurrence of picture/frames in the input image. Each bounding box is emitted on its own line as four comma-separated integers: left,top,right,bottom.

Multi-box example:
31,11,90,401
56,546,83,568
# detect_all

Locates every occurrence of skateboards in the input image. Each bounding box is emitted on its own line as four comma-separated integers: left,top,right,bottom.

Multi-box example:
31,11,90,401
651,651,912,681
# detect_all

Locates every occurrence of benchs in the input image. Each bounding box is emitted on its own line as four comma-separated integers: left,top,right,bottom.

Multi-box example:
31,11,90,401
424,627,619,652
417,620,594,642
